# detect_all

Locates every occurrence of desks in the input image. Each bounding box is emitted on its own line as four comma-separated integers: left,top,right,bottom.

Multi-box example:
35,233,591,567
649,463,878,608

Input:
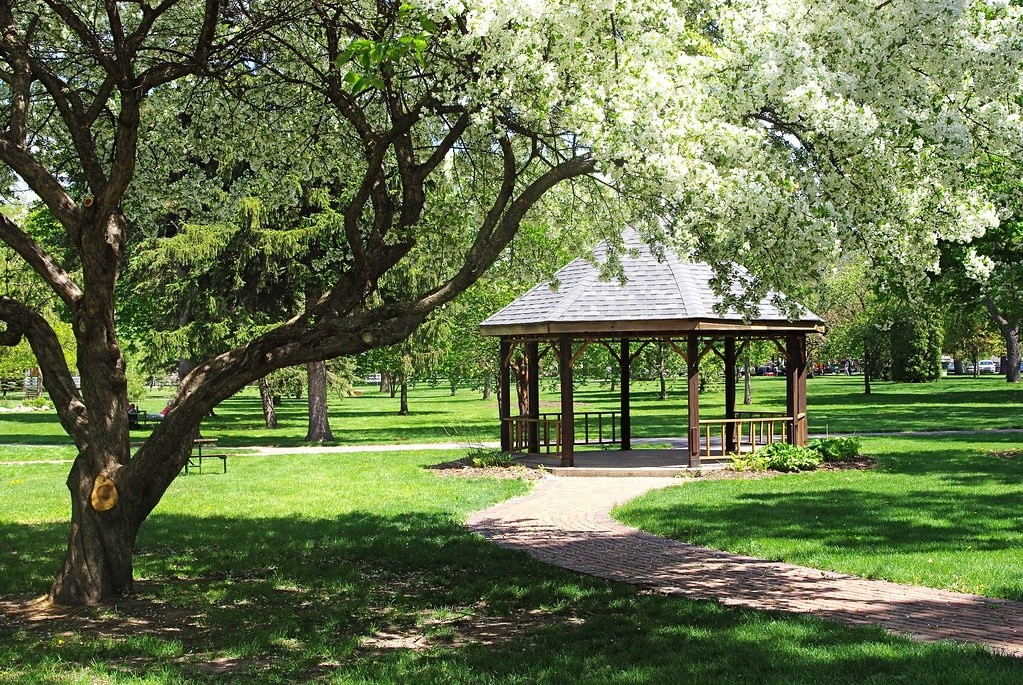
186,438,218,474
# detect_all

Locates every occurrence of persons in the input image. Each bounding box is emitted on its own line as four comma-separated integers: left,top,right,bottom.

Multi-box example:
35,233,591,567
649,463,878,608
145,397,177,420
127,403,135,413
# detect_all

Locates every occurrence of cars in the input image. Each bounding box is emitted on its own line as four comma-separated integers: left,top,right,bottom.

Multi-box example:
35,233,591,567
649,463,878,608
947,361,956,374
976,360,997,373
995,365,1001,372
1019,361,1023,372
963,364,975,374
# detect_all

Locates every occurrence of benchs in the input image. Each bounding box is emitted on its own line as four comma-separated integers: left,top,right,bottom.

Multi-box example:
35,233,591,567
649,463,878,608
187,454,227,474
